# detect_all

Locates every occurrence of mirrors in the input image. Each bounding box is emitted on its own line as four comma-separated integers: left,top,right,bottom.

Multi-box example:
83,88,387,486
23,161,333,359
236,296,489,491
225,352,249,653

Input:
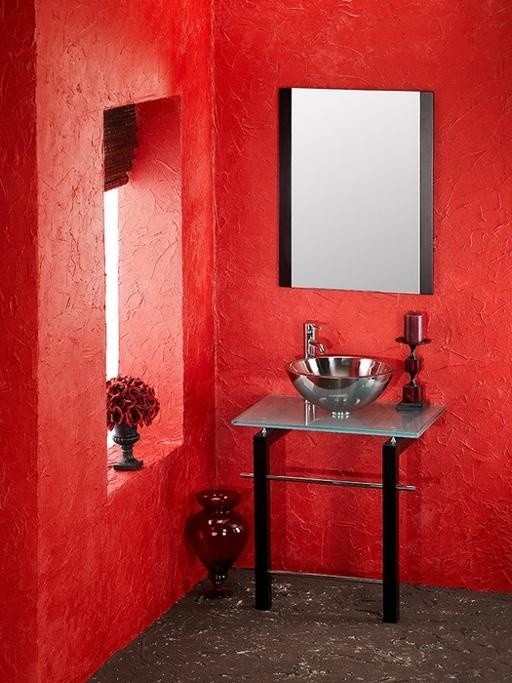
278,86,435,295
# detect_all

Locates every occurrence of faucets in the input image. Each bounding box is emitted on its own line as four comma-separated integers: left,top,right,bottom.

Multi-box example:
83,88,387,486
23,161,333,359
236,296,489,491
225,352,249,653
305,396,320,427
302,319,326,402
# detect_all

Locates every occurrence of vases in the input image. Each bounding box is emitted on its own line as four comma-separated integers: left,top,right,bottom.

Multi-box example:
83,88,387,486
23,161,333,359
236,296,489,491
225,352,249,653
186,489,248,599
111,422,144,471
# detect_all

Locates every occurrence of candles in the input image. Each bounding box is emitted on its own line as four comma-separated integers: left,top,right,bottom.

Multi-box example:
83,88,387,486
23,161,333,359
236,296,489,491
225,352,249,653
404,313,424,344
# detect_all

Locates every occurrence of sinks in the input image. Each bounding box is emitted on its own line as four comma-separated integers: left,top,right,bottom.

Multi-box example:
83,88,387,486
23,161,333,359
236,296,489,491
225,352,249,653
285,352,393,412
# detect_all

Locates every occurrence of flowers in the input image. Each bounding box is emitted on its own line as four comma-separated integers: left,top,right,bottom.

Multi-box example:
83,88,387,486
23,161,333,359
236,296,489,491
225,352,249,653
106,375,162,432
